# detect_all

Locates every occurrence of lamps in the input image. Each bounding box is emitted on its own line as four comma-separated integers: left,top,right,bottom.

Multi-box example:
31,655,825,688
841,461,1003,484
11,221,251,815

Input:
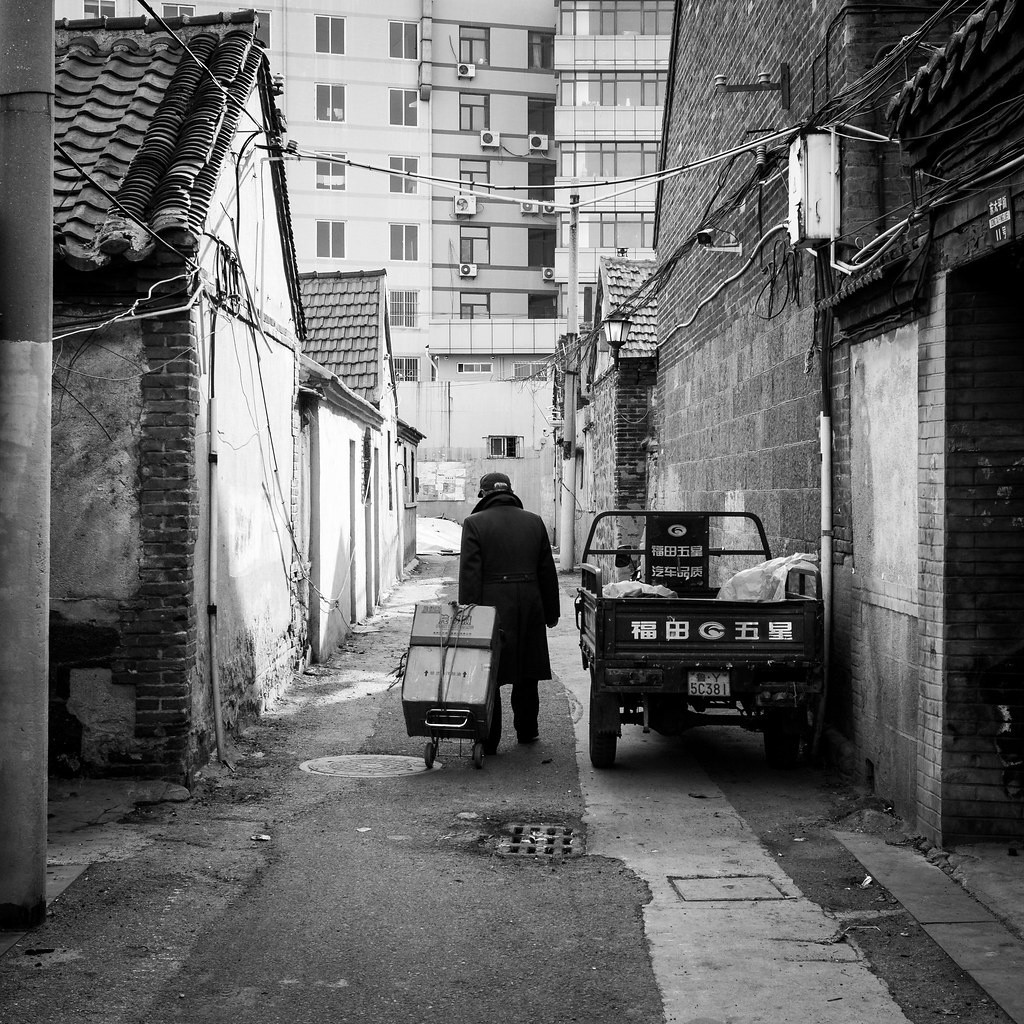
602,302,633,372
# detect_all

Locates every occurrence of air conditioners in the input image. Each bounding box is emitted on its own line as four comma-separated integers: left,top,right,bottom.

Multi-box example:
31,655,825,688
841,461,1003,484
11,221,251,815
527,134,548,150
542,267,555,281
542,200,555,214
459,264,477,277
480,130,500,147
453,195,476,215
520,200,539,213
457,63,475,77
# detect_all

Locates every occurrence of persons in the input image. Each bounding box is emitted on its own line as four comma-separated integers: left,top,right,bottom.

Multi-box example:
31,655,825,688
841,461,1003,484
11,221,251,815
458,473,561,753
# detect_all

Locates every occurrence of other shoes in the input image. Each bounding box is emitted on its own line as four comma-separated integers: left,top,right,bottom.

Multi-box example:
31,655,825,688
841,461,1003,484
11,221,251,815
517,737,539,743
471,744,497,756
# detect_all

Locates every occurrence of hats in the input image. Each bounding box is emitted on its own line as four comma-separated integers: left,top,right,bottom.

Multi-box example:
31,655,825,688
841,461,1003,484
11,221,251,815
477,472,512,498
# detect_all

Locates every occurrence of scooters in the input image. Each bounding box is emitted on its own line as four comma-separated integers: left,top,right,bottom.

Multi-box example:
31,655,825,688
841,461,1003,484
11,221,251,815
576,511,822,771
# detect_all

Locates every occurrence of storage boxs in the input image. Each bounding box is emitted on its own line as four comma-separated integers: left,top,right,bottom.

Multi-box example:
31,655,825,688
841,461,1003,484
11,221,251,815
400,603,500,738
